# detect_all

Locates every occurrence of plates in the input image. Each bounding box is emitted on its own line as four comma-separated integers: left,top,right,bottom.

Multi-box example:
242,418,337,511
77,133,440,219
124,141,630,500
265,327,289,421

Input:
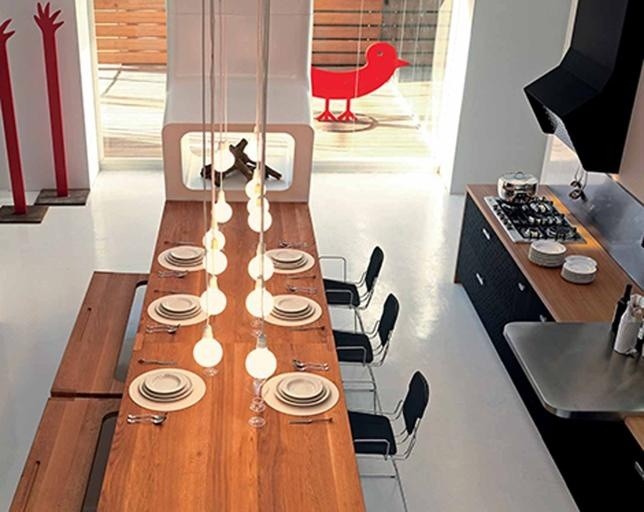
529,240,599,284
266,249,315,274
128,368,207,411
158,246,205,272
261,372,340,416
147,293,209,327
266,294,323,327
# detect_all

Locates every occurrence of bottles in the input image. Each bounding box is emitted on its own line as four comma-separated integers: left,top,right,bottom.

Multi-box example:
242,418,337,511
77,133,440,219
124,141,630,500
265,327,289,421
612,283,642,354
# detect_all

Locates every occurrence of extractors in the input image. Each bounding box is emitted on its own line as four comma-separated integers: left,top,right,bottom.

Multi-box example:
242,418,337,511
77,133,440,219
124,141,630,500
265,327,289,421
522,0,643,174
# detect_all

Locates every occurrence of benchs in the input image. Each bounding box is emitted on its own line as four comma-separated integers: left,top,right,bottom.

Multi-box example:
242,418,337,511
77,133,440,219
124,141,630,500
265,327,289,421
5,269,151,512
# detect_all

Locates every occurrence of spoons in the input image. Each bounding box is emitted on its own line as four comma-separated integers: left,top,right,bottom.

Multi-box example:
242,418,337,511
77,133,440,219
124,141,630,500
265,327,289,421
126,411,168,425
287,284,317,294
145,323,180,334
158,270,188,278
291,359,330,372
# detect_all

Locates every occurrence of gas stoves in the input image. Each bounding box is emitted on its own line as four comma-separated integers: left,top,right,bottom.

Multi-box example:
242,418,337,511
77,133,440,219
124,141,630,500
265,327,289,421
484,195,587,244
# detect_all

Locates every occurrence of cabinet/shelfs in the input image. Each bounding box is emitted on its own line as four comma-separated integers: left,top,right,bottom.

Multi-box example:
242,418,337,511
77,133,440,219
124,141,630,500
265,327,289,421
494,263,644,512
456,194,514,333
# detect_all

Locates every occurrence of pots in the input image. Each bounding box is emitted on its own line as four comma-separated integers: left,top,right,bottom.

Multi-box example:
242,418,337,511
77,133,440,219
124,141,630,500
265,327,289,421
498,170,538,204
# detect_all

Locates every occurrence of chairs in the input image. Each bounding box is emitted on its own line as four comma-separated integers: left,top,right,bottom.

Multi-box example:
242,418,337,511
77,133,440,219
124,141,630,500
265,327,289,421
317,246,430,512
